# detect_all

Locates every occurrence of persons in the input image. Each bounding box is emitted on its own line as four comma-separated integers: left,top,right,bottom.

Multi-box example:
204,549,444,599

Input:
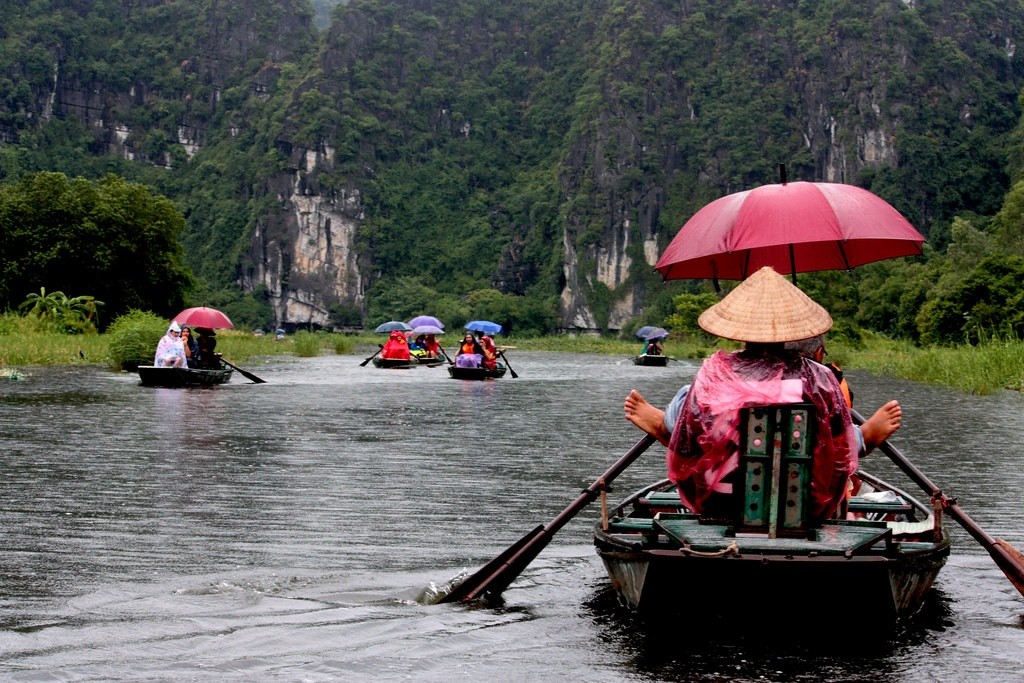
455,331,497,371
153,321,218,369
382,331,442,360
624,266,902,531
640,338,663,356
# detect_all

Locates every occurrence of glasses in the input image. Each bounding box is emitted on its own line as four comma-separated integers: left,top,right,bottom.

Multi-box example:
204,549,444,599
823,350,828,358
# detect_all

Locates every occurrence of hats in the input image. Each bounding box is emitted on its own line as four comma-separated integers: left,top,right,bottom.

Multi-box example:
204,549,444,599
194,327,216,336
698,266,833,342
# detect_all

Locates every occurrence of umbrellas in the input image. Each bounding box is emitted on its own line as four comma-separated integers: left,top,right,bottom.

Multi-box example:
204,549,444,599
651,162,927,287
374,316,445,333
170,302,235,329
193,327,216,336
463,321,502,335
635,326,670,340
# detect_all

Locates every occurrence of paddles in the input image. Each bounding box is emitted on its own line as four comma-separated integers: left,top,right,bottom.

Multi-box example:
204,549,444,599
499,351,519,379
850,407,1024,595
358,348,383,366
220,357,267,384
377,343,425,364
433,433,656,606
669,357,678,362
437,343,453,365
628,353,637,360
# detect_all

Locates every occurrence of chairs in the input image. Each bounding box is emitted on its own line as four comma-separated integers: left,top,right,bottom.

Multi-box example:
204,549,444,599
650,402,893,556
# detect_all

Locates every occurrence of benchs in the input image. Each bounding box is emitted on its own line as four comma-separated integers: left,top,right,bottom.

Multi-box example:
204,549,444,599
609,513,653,529
647,489,910,514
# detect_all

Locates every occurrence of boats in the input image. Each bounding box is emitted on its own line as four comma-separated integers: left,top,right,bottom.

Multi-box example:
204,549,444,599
593,474,951,641
372,350,446,369
447,359,506,380
138,362,236,388
633,352,668,366
